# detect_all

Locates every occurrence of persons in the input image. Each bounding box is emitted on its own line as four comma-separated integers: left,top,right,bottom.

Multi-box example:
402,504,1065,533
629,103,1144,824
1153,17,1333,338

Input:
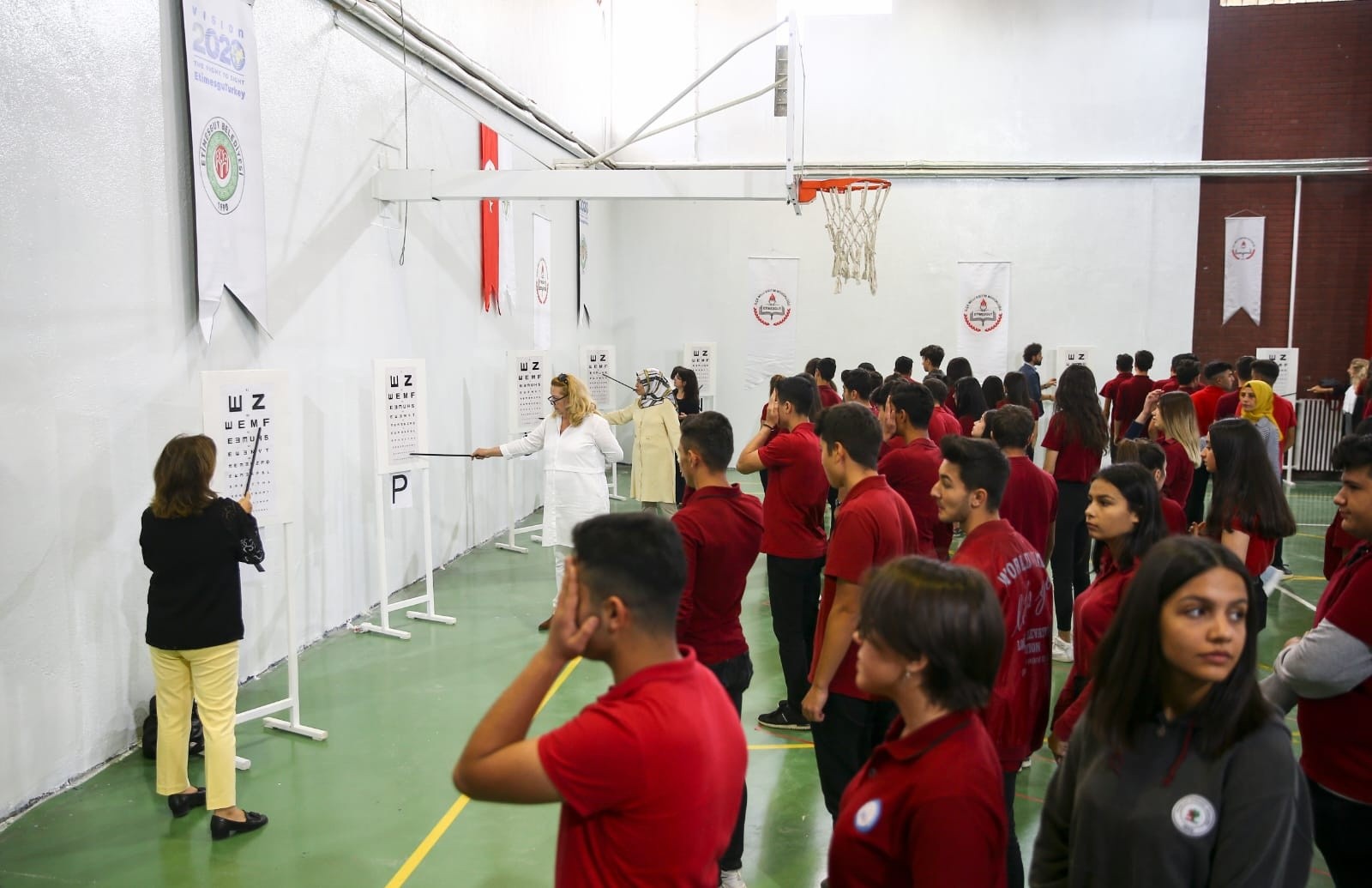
596,369,687,520
451,505,747,888
666,367,700,509
135,434,272,840
762,340,1300,559
1257,429,1372,888
799,401,922,827
1185,415,1298,666
735,376,829,730
1309,358,1370,435
1042,362,1110,662
1045,462,1169,769
658,413,766,884
1027,534,1316,888
823,553,1012,888
470,373,627,631
930,436,1054,888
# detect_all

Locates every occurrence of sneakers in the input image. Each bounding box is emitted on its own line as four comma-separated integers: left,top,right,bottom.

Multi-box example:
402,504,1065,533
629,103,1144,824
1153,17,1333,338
1051,636,1075,663
717,870,746,888
778,700,789,708
1276,567,1294,580
758,705,812,731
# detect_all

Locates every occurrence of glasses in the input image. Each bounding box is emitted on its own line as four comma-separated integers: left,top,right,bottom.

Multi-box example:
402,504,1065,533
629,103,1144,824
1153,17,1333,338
548,394,569,406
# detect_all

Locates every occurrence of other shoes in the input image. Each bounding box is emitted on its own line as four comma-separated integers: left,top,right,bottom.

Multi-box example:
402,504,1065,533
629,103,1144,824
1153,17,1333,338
539,615,554,630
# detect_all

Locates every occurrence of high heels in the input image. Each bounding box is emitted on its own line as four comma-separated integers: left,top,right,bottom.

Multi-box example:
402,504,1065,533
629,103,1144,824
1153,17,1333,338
211,809,268,839
168,787,207,818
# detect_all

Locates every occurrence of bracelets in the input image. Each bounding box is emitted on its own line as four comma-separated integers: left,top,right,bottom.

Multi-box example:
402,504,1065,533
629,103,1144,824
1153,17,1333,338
763,423,775,432
